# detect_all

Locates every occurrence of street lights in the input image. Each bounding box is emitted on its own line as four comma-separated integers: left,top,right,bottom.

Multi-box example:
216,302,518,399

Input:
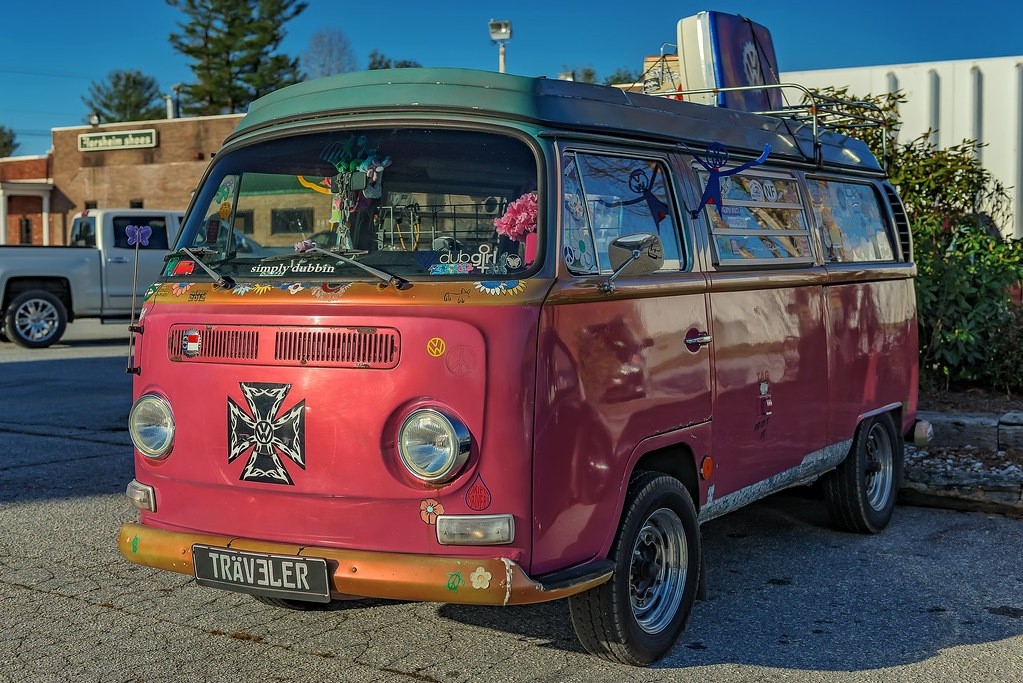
488,20,512,78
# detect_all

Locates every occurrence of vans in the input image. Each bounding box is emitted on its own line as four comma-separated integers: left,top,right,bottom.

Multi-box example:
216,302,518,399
116,64,919,668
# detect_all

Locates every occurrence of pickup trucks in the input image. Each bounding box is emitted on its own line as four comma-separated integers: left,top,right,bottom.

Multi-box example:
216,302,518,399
0,209,284,349
